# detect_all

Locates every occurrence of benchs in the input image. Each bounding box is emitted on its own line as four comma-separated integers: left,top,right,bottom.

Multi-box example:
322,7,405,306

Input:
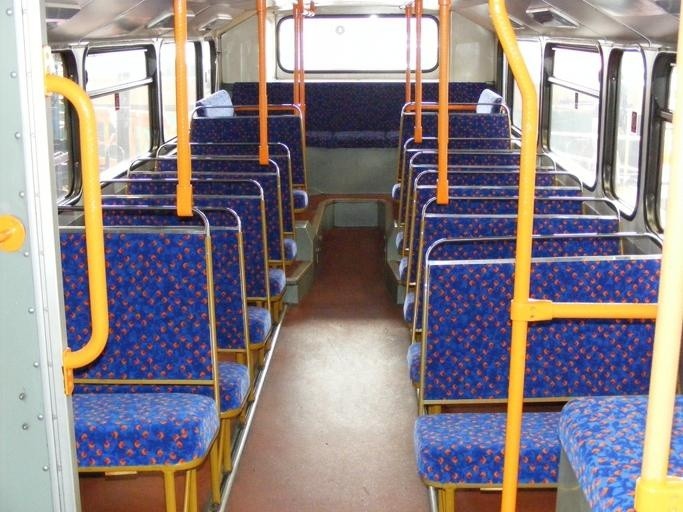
404,190,629,391
120,81,314,356
410,229,669,512
58,203,226,510
85,197,260,506
545,384,683,512
386,81,587,320
